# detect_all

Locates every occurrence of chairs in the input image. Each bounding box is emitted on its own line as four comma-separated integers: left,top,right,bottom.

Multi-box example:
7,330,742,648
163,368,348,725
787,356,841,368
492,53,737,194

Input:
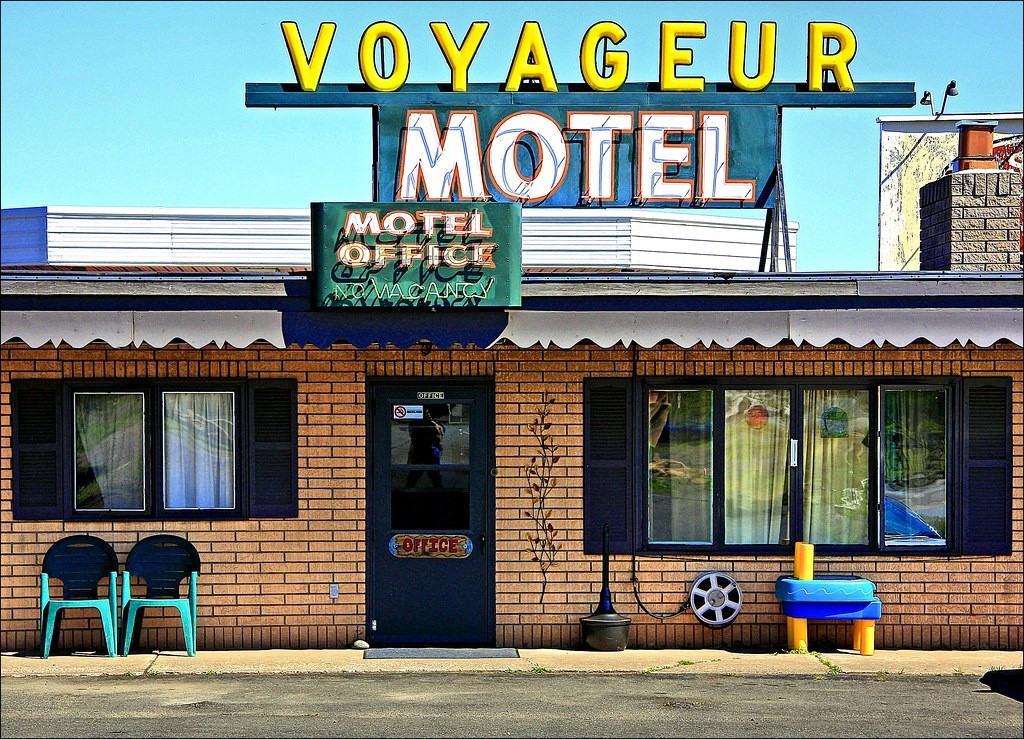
40,535,118,658
120,535,201,656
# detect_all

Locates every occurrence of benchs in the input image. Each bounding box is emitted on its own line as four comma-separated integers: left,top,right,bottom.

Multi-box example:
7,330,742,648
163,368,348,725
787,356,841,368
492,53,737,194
775,541,881,652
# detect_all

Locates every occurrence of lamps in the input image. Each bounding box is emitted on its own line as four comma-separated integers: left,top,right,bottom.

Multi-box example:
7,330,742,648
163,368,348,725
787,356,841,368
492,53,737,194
920,90,936,116
936,80,959,115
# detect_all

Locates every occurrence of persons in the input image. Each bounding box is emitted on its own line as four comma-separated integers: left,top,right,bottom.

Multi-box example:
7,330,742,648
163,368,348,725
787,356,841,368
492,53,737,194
405,403,446,465
